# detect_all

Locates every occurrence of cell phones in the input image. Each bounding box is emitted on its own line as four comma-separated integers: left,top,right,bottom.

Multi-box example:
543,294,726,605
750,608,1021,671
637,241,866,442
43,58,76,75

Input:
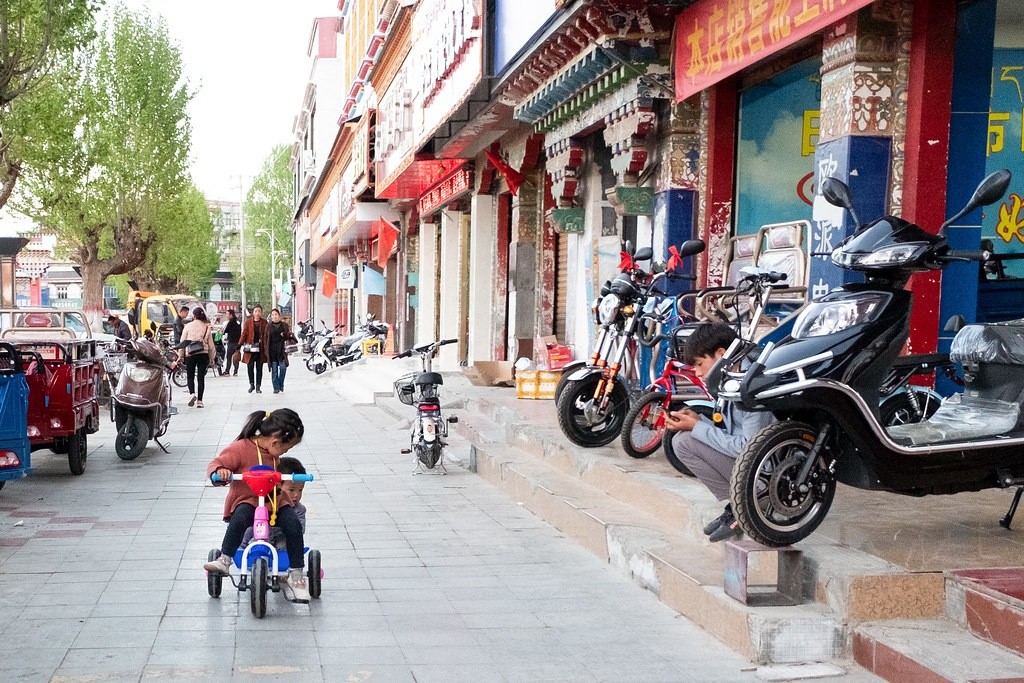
658,405,679,422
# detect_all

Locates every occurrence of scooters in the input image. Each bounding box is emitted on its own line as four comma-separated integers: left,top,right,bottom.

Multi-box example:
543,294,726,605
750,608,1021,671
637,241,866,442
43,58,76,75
210,327,225,377
728,168,1024,549
296,312,390,374
392,339,459,476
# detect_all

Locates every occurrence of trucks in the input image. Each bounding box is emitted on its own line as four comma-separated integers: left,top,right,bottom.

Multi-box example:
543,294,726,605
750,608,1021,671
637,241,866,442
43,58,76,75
127,293,224,349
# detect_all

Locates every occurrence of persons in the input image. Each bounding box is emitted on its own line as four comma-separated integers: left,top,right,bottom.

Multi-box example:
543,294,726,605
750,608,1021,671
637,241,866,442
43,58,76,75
662,321,778,543
235,304,268,393
174,306,189,347
220,309,241,376
238,456,307,549
204,408,305,581
297,321,309,343
108,316,131,346
264,308,290,393
178,307,217,408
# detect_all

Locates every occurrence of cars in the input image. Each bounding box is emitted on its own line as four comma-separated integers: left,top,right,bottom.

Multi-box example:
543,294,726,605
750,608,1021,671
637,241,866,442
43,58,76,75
0,306,117,357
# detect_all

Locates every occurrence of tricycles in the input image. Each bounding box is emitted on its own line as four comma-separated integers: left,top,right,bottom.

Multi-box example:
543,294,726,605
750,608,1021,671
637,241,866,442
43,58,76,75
208,465,325,619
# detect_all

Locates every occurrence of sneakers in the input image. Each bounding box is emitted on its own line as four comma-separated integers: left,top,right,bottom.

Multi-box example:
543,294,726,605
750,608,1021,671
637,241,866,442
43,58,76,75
203,555,229,577
708,512,741,542
704,504,733,534
288,567,306,589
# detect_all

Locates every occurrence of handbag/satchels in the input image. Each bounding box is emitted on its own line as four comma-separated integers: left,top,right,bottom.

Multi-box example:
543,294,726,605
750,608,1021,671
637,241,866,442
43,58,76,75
232,350,241,364
189,342,204,354
243,344,259,353
284,339,298,353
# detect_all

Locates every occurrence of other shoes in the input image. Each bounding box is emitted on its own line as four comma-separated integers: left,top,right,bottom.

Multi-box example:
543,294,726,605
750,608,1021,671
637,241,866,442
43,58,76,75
281,385,283,392
233,373,238,376
273,390,279,393
187,393,197,407
248,384,255,393
197,400,204,408
223,372,229,376
256,386,262,393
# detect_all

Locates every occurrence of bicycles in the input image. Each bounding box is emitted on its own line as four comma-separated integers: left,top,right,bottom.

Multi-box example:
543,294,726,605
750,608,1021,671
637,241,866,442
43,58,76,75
620,269,779,459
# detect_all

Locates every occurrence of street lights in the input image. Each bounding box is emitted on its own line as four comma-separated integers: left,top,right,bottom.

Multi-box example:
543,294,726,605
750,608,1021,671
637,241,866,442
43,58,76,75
254,229,277,310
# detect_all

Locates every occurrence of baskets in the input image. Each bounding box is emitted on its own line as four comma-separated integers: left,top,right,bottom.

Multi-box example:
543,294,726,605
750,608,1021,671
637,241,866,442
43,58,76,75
103,353,128,373
394,371,421,404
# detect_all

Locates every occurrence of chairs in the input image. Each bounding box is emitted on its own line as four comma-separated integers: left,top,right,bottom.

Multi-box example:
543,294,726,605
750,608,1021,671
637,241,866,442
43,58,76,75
675,218,812,327
462,336,571,400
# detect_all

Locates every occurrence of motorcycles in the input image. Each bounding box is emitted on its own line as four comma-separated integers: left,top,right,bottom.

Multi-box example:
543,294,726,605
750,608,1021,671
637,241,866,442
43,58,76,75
555,219,818,447
0,309,100,491
109,321,188,461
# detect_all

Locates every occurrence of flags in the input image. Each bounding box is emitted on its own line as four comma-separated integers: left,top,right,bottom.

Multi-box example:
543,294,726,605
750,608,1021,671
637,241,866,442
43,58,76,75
484,151,526,196
321,271,336,298
378,217,400,268
277,293,291,307
